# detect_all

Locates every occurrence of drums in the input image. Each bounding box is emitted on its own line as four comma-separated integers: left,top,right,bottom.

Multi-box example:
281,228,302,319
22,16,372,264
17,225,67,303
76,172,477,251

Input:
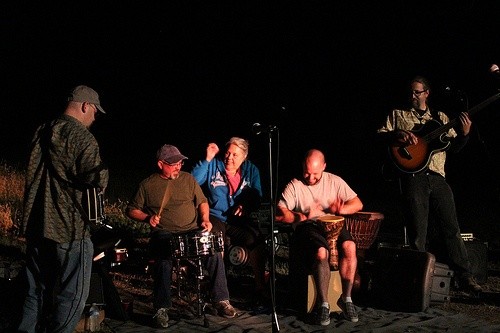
168,231,225,261
344,212,385,291
97,249,129,267
317,217,349,270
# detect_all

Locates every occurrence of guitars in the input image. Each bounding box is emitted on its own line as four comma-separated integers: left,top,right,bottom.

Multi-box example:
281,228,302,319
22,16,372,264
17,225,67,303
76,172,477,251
85,186,104,226
389,94,498,174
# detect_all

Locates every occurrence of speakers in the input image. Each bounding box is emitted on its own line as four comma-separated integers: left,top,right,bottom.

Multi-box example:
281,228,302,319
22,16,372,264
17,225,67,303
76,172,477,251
366,247,453,313
428,235,488,283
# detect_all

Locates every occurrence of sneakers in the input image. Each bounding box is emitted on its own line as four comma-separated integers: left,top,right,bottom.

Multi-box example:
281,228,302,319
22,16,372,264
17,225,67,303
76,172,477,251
336,295,358,323
213,299,238,318
153,308,169,328
318,303,331,325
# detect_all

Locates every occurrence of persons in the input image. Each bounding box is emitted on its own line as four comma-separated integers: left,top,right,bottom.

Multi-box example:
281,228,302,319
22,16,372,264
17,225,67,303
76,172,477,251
125,144,238,328
16,85,107,333
190,136,272,300
375,75,483,299
275,149,364,325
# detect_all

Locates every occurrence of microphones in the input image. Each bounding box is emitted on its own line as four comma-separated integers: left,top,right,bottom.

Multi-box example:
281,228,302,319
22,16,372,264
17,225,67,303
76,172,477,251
252,123,278,135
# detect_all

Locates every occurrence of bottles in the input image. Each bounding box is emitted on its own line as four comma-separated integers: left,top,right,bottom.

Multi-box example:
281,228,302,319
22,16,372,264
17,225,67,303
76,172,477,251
89,303,100,333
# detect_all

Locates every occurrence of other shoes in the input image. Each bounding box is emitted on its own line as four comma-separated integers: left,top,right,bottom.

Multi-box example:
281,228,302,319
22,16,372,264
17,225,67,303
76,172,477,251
450,270,483,298
253,289,270,311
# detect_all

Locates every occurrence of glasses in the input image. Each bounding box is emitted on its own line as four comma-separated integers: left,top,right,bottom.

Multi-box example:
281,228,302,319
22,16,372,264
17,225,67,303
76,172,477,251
161,161,184,166
89,104,99,116
408,89,428,97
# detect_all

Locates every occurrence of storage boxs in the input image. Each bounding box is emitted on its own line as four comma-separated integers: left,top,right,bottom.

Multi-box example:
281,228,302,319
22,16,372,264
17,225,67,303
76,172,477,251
372,245,454,312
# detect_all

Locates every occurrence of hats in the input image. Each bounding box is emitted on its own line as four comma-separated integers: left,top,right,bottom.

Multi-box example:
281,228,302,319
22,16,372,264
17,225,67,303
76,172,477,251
157,144,188,163
68,85,106,113
